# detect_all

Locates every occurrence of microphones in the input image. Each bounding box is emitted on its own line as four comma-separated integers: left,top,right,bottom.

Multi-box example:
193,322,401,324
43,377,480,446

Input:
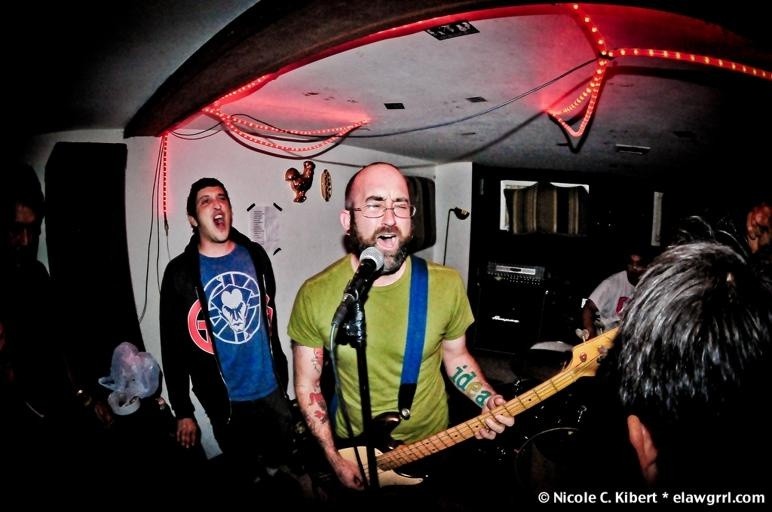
331,247,384,328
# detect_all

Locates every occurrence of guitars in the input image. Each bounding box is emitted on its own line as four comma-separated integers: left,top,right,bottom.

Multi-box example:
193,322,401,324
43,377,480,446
308,326,621,510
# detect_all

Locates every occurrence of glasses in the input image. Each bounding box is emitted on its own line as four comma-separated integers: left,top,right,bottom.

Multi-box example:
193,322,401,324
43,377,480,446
346,203,418,221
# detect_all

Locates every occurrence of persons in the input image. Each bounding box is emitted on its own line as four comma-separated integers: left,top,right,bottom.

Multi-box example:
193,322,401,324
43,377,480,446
285,162,517,512
158,175,288,509
580,249,650,343
1,154,65,510
605,209,772,510
744,204,771,254
